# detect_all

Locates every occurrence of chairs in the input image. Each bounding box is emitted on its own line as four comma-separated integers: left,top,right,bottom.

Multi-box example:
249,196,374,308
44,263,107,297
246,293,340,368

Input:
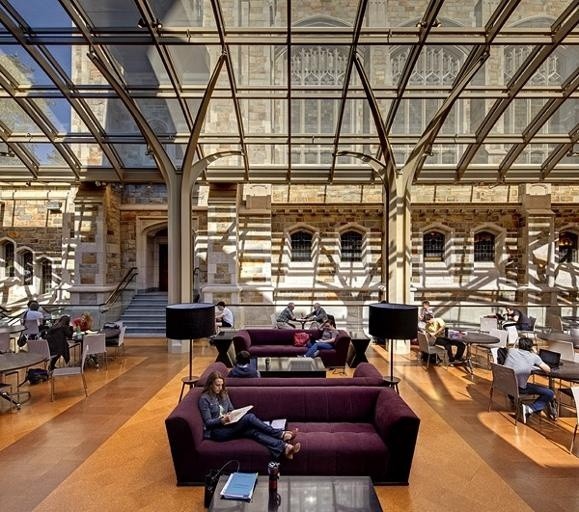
0,317,129,426
407,307,579,453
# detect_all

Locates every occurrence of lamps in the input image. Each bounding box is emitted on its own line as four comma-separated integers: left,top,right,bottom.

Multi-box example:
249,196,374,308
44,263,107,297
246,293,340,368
164,300,220,402
366,302,418,392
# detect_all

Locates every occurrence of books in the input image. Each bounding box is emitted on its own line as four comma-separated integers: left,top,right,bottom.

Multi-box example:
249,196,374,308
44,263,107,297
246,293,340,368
219,471,257,500
225,404,256,425
270,418,287,430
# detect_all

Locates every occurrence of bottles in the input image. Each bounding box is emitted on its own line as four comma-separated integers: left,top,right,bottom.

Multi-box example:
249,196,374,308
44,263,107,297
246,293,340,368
268,490,278,512
268,466,277,490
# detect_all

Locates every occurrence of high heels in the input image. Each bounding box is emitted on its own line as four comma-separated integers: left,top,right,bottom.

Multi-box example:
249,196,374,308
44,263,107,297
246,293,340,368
282,428,300,459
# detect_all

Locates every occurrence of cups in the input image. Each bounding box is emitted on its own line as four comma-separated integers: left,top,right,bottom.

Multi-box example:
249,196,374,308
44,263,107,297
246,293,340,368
445,327,448,335
77,326,80,333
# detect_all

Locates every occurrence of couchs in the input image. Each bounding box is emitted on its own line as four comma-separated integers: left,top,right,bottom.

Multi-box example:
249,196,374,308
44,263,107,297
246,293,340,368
163,383,416,484
196,326,386,385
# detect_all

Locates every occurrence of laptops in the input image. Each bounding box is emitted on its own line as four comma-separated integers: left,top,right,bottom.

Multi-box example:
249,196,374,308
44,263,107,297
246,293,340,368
534,349,561,369
496,313,510,320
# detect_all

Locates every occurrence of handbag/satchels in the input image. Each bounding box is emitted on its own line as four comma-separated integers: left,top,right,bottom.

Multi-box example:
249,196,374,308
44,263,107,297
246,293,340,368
28,368,49,384
294,333,310,347
17,336,27,347
205,460,239,508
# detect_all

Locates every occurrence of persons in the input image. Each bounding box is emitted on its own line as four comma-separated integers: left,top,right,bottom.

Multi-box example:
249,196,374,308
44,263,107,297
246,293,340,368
305,302,328,331
503,305,531,331
503,337,554,425
277,302,297,329
23,300,99,372
214,301,233,328
417,301,466,366
227,350,262,378
296,319,337,359
198,370,301,460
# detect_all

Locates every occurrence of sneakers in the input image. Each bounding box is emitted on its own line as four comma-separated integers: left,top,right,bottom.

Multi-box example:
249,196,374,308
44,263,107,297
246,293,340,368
521,404,531,424
